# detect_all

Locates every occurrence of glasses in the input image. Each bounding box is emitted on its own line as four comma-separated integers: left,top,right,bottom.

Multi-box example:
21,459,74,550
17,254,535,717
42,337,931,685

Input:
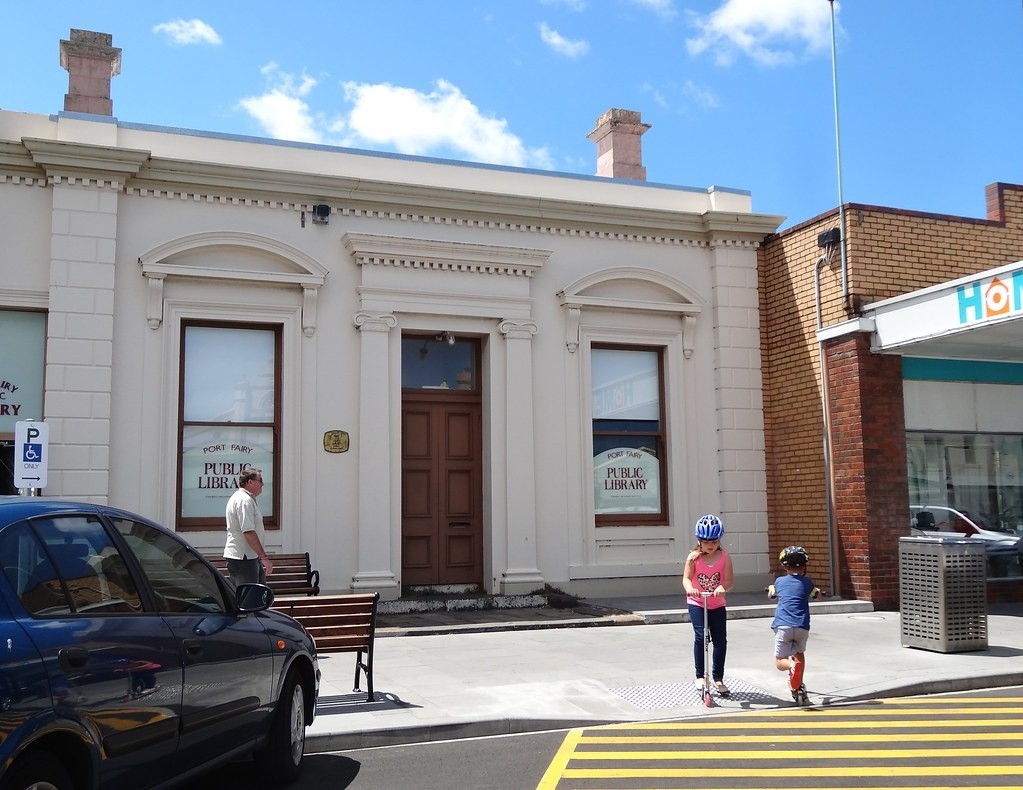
246,477,263,484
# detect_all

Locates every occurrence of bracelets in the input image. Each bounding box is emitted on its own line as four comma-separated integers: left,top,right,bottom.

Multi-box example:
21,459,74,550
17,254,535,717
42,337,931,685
259,557,268,560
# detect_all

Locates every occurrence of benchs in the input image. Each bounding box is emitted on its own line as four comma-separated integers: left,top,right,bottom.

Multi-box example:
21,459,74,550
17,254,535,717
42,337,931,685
266,591,381,702
206,552,320,600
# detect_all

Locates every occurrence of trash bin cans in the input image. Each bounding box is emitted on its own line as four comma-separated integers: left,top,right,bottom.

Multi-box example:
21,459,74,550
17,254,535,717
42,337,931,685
898,534,991,654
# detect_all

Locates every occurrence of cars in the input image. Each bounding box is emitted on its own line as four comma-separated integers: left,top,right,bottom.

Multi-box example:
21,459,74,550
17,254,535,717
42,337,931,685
907,506,1023,559
0,494,321,790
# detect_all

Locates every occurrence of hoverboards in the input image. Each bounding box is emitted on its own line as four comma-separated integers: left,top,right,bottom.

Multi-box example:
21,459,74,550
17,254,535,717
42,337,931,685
687,588,730,707
764,586,826,705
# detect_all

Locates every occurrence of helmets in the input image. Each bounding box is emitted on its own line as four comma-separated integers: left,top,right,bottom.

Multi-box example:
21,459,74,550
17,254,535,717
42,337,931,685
778,546,809,569
694,514,724,540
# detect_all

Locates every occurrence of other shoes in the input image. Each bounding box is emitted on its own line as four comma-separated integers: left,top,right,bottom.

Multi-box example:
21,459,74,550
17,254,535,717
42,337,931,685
712,679,728,693
792,683,806,700
790,662,803,689
694,677,705,690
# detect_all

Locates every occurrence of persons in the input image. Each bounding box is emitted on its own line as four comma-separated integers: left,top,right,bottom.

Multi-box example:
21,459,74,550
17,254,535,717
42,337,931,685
682,514,734,690
222,466,273,588
767,545,821,690
